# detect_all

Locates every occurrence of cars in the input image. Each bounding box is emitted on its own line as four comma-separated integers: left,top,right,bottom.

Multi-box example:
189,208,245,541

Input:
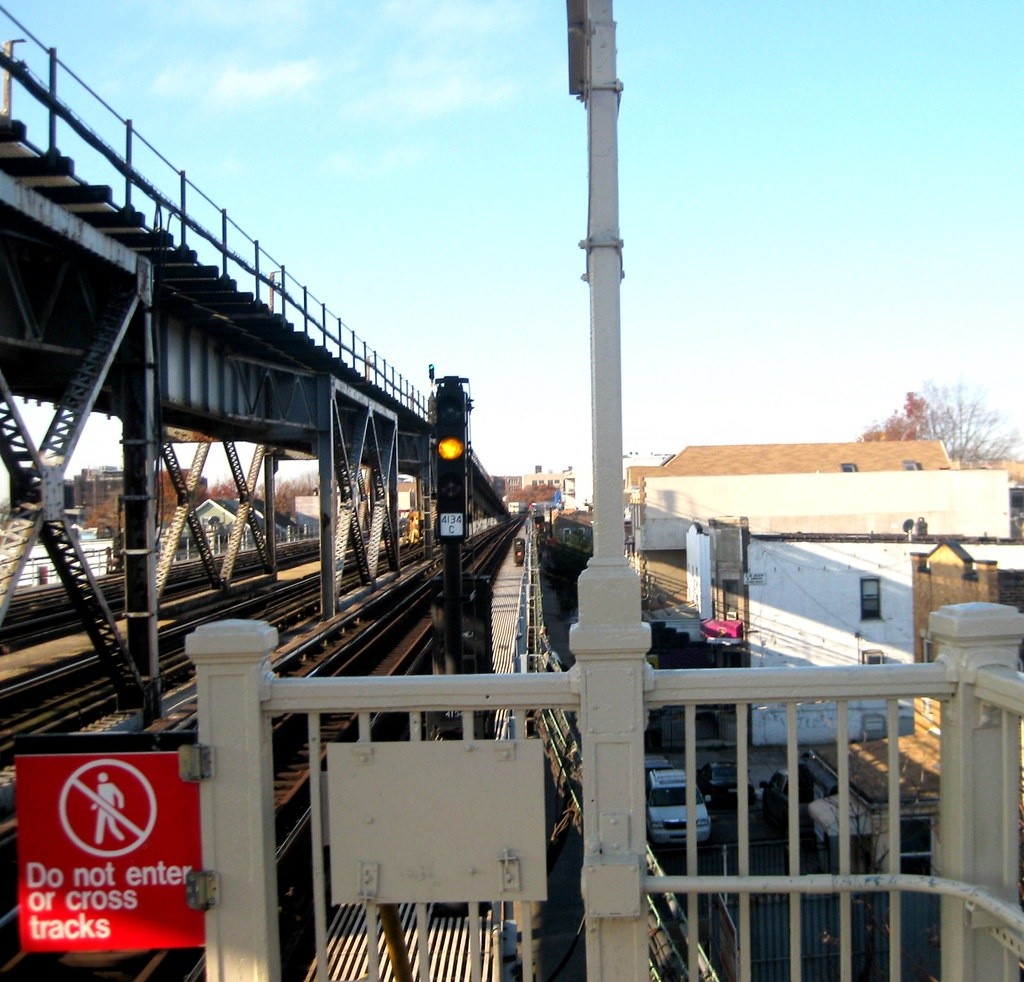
695,761,756,812
760,768,788,814
644,753,676,784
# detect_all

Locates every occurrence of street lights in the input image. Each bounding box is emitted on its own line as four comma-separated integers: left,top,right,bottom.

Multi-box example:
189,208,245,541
550,506,557,538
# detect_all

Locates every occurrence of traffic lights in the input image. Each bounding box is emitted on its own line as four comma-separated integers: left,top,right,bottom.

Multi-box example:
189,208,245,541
435,374,470,540
428,363,435,380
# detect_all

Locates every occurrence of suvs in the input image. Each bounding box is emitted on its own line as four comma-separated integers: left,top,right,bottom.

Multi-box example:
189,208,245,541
647,768,712,846
